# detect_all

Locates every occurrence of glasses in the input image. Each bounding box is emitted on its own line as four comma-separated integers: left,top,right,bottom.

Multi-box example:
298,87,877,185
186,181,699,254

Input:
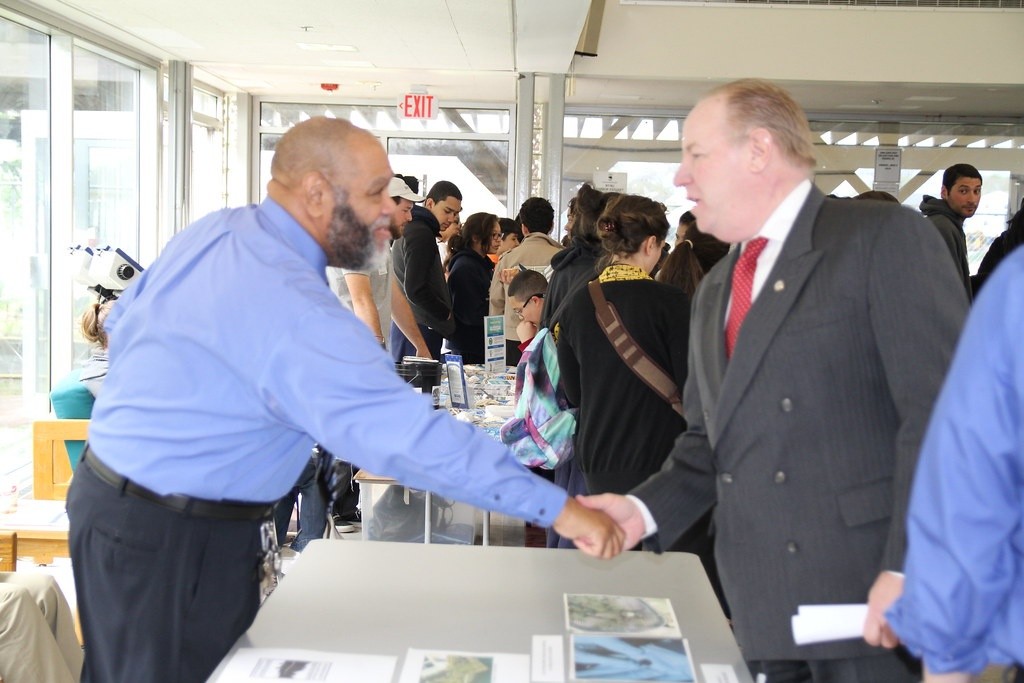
489,232,505,240
452,218,461,225
514,293,545,317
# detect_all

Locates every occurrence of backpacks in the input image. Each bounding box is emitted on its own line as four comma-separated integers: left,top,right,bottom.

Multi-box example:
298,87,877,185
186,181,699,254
500,265,600,470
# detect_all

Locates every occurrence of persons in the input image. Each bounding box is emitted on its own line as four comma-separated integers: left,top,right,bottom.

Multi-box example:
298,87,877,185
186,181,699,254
570,78,973,683
545,194,693,553
64,114,624,683
884,246,1024,683
0,570,84,683
648,210,729,296
971,195,1023,299
919,163,982,295
287,174,621,552
78,300,117,396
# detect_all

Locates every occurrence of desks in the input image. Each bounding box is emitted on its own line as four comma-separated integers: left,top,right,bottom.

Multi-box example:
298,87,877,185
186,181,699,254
350,360,521,544
0,496,72,563
202,539,752,683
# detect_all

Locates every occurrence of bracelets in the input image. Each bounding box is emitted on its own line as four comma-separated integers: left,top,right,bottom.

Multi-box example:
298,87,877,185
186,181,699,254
381,338,386,349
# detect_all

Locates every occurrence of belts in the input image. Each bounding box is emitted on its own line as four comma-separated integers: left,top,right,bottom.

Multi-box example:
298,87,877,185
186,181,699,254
84,442,281,524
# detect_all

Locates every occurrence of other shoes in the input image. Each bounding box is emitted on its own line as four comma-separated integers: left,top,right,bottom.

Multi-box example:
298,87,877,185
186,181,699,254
333,519,357,532
341,509,363,528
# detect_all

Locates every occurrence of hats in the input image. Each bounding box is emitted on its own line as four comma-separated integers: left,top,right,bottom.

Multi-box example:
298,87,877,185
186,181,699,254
387,177,426,203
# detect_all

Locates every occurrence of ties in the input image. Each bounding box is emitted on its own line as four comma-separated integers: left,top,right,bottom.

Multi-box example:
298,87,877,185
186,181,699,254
725,237,769,363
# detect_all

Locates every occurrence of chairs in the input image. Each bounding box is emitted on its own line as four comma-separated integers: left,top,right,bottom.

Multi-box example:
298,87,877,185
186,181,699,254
31,386,97,501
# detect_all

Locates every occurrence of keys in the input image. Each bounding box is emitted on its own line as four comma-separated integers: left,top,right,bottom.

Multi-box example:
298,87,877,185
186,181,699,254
256,548,284,603
